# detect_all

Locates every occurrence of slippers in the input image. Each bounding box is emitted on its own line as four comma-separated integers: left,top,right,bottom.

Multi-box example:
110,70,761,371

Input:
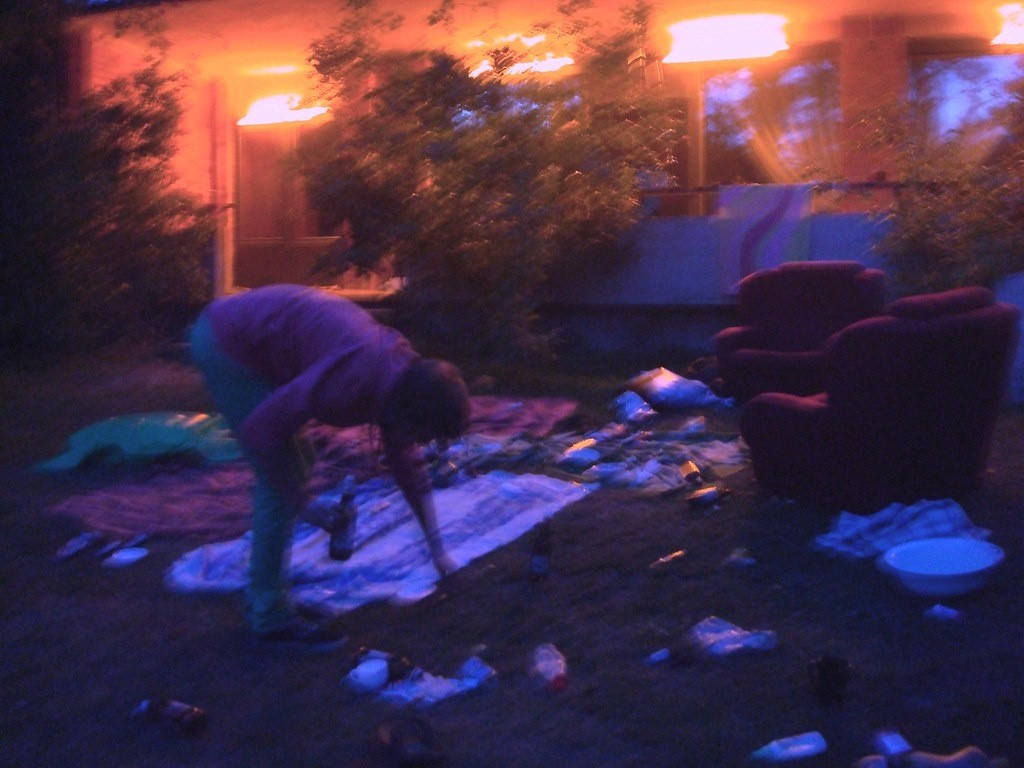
375,714,445,766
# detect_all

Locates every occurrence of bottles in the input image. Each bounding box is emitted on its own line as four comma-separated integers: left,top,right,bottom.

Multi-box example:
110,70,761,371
148,696,207,730
870,725,912,768
535,640,568,690
356,648,422,682
745,732,828,763
678,459,702,487
650,549,689,570
685,487,731,504
646,616,780,662
528,514,551,582
328,482,358,561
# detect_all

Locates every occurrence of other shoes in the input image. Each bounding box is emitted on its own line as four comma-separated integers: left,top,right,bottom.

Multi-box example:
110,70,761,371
258,616,324,641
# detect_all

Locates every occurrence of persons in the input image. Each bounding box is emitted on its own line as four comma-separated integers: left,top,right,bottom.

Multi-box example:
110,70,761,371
190,282,471,655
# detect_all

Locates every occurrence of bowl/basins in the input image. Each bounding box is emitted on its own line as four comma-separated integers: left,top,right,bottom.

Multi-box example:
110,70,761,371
883,540,1005,599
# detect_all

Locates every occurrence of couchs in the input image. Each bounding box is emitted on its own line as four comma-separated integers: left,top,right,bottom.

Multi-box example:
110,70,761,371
713,259,886,406
734,287,1020,508
